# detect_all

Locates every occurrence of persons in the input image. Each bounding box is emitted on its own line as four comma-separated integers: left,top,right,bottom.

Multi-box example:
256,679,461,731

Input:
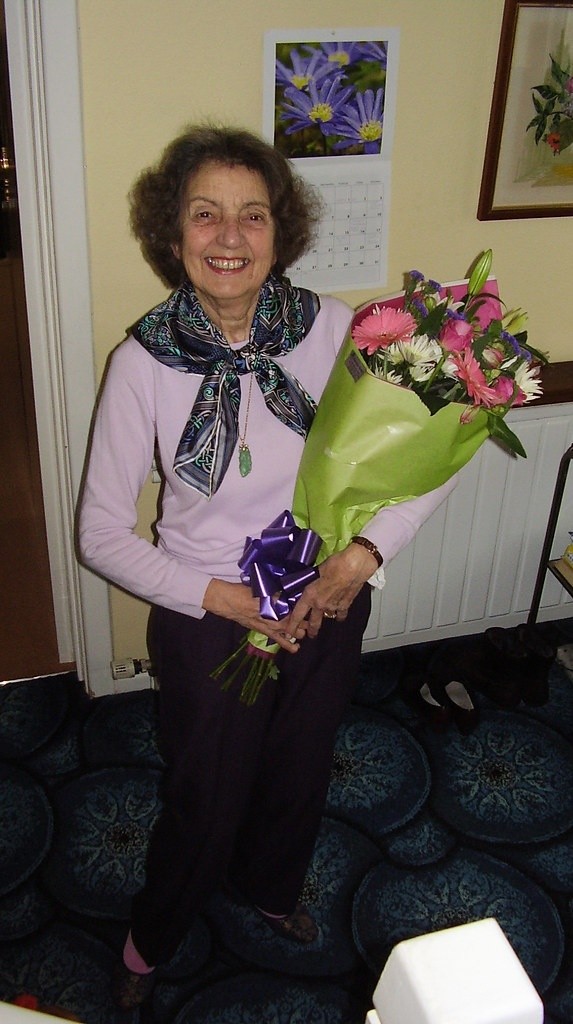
78,125,458,1024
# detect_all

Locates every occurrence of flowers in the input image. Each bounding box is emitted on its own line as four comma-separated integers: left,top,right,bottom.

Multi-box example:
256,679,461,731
207,249,551,706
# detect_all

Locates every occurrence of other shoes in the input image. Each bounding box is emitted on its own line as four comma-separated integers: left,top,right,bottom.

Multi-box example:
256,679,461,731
230,880,319,943
114,921,164,1010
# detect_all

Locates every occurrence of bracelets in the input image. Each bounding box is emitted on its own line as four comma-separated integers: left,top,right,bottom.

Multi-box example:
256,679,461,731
350,535,383,569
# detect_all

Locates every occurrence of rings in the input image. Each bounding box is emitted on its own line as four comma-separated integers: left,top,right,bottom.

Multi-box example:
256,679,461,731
324,611,336,620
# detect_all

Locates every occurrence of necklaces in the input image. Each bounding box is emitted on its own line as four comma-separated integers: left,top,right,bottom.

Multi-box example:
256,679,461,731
239,371,254,477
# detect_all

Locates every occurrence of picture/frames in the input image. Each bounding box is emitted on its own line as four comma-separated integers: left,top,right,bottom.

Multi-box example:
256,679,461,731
475,0,573,221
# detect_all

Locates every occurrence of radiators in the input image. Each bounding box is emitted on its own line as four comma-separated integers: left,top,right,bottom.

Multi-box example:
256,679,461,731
360,402,572,654
